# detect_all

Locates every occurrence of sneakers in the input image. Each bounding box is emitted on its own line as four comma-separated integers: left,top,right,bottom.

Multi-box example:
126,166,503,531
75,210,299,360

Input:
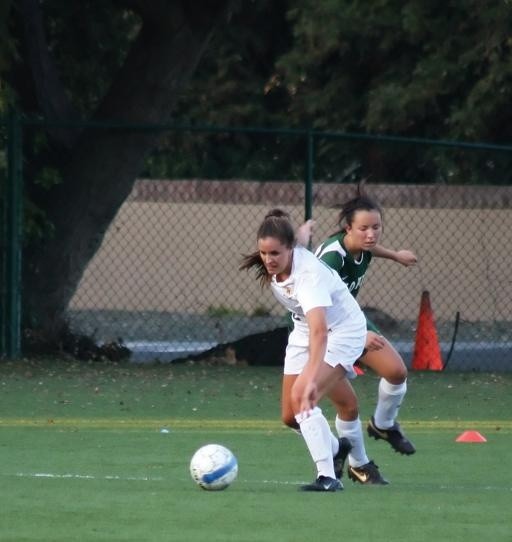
367,415,416,456
300,474,344,493
348,460,389,486
333,436,353,479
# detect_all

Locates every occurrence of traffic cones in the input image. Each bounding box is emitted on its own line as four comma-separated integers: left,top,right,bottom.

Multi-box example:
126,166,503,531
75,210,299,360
409,290,445,373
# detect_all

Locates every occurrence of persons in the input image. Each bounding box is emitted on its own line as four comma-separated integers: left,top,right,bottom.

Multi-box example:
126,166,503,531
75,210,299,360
238,204,370,494
308,195,420,488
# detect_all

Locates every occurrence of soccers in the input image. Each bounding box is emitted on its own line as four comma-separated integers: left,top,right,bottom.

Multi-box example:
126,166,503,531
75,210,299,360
190,445,238,491
